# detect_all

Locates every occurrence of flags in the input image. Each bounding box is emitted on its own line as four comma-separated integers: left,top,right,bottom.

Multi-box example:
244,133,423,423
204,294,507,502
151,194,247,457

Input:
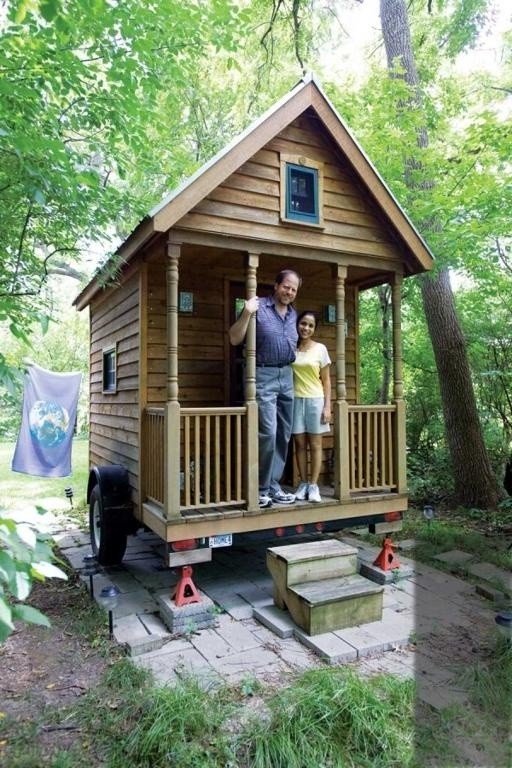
11,364,83,479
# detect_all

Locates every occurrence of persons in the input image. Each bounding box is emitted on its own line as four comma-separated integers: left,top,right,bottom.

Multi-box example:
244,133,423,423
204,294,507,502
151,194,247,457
228,269,303,509
288,309,333,504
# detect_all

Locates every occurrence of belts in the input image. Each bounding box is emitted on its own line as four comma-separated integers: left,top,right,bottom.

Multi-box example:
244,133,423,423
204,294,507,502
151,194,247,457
256,362,290,368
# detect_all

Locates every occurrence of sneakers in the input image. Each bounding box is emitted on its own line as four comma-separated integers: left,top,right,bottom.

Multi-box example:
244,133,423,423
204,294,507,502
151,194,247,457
293,482,308,500
308,484,322,504
270,489,296,504
258,495,272,508
